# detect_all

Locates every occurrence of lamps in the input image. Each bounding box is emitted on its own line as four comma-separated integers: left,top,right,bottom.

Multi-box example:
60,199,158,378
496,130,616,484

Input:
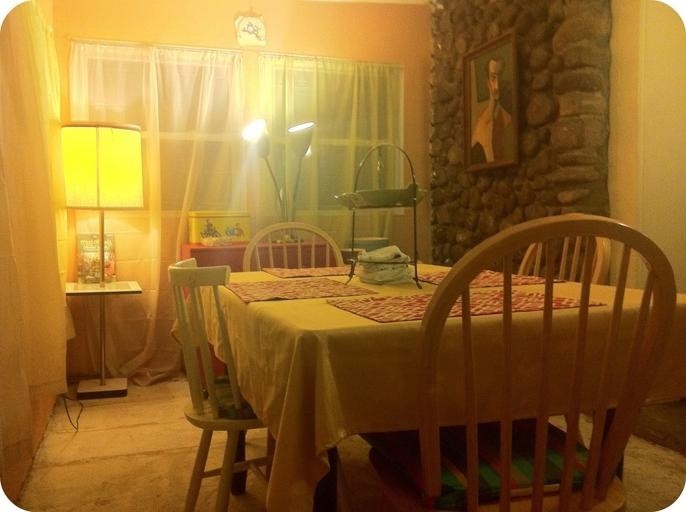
59,122,150,403
241,117,316,242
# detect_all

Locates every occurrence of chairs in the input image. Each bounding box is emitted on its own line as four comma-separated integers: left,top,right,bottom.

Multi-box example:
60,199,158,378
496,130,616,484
166,259,277,510
339,219,678,512
240,218,346,270
512,213,615,454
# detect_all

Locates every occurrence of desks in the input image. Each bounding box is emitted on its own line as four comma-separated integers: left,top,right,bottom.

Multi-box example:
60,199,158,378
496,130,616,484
184,260,685,508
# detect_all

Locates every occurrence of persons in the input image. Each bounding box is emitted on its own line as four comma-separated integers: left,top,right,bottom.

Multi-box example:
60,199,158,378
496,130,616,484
471,55,512,164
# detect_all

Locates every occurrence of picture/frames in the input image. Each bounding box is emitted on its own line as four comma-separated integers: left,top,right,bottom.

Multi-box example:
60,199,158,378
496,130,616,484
460,28,515,176
